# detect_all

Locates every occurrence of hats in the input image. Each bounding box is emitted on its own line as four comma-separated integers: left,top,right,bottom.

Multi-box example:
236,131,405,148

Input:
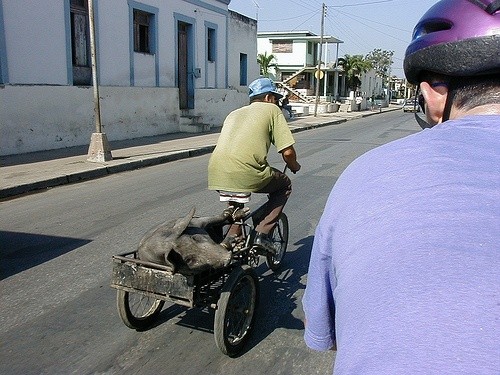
248,77,284,100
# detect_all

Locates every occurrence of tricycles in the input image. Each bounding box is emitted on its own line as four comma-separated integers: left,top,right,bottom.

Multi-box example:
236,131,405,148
109,164,291,357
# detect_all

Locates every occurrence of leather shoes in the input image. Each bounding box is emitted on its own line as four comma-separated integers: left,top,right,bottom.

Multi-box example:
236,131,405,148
253,235,277,256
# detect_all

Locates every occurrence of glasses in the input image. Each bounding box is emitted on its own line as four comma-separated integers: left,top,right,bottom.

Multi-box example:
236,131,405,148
419,83,447,114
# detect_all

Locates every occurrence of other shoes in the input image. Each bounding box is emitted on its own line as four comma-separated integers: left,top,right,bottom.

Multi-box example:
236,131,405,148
290,116,295,120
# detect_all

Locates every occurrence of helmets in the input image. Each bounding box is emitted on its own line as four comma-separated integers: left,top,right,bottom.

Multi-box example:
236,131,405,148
403,0,500,85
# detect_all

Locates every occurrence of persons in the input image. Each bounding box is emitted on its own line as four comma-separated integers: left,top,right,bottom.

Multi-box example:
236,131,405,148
282,95,295,120
303,1,500,375
207,77,301,256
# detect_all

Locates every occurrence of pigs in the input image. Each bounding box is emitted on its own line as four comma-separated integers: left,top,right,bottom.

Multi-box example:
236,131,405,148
137,206,238,277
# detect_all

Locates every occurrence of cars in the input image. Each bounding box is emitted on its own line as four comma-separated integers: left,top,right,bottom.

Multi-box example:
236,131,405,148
403,100,420,113
